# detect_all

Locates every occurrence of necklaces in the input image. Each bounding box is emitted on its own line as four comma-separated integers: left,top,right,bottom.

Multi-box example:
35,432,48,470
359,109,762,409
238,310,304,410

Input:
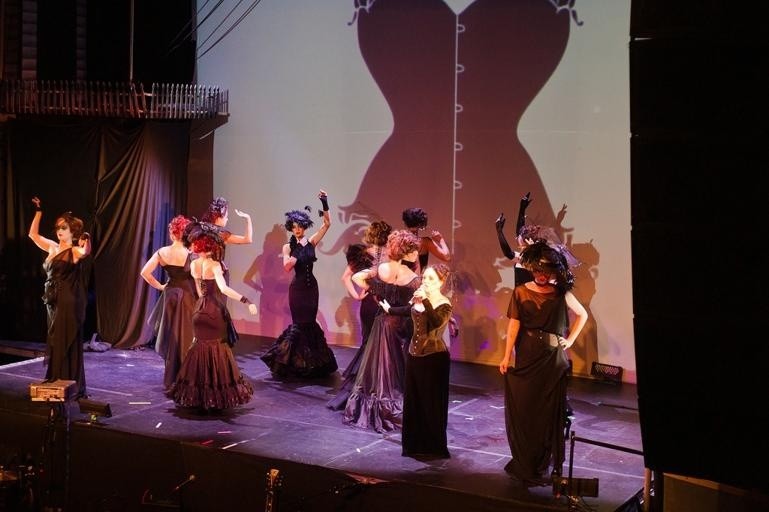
534,279,550,287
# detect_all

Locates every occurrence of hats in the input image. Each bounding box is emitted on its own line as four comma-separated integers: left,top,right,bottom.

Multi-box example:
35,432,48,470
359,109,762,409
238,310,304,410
286,210,310,226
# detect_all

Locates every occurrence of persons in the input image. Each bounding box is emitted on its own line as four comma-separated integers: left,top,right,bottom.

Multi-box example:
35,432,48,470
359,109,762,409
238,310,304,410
495,191,562,352
498,240,590,484
341,207,458,458
28,196,93,401
282,189,332,374
139,195,258,411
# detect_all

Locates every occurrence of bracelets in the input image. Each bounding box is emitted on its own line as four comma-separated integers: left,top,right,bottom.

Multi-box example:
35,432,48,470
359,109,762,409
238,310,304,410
35,206,42,211
